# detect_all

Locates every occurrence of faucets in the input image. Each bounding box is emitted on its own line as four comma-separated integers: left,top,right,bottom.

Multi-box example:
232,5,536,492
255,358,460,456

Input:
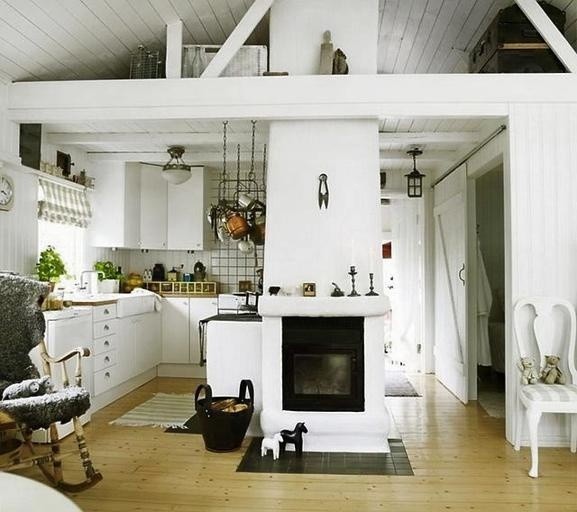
78,270,106,288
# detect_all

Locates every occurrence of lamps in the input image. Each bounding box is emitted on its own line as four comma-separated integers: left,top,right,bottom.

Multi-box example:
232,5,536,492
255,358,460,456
161,147,192,185
404,150,425,197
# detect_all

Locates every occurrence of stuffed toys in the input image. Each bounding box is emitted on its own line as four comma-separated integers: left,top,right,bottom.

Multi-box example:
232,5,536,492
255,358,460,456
541,354,566,384
517,357,541,385
2,375,55,400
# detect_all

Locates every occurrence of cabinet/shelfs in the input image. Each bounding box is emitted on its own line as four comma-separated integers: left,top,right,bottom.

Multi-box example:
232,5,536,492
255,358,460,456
74,294,162,412
158,294,218,379
94,162,210,251
25,307,93,443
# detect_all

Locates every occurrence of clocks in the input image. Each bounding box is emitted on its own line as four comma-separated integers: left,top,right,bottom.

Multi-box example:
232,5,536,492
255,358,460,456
0,174,14,210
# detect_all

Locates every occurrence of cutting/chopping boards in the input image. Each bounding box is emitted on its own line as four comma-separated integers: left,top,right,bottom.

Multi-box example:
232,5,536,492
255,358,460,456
72,299,117,305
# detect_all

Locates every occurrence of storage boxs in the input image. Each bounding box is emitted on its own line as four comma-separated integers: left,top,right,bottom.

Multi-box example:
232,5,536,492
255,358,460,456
184,43,267,77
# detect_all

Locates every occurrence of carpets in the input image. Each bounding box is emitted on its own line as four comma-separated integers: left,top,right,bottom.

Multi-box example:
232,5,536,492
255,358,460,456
385,372,419,397
478,390,505,418
109,391,207,428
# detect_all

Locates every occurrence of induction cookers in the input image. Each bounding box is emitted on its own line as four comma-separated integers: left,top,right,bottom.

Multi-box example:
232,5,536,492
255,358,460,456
200,313,262,324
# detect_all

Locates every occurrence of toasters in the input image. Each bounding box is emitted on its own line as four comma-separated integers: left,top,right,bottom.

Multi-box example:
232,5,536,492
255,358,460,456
80,271,106,295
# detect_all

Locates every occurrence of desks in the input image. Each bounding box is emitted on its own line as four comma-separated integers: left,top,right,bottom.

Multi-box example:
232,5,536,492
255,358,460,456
258,295,389,454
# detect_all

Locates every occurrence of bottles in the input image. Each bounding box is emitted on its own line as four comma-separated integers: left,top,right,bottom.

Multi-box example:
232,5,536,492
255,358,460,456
143,261,206,282
225,213,250,240
115,266,123,293
182,47,203,77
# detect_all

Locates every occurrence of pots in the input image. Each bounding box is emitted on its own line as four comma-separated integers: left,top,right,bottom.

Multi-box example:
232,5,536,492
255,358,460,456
251,219,265,245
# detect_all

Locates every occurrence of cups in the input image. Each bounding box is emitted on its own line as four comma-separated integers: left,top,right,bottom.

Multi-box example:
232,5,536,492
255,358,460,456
49,291,64,310
238,194,252,207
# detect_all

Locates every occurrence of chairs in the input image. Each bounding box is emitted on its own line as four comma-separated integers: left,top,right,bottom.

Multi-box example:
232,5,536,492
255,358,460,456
0,274,102,492
513,297,577,478
